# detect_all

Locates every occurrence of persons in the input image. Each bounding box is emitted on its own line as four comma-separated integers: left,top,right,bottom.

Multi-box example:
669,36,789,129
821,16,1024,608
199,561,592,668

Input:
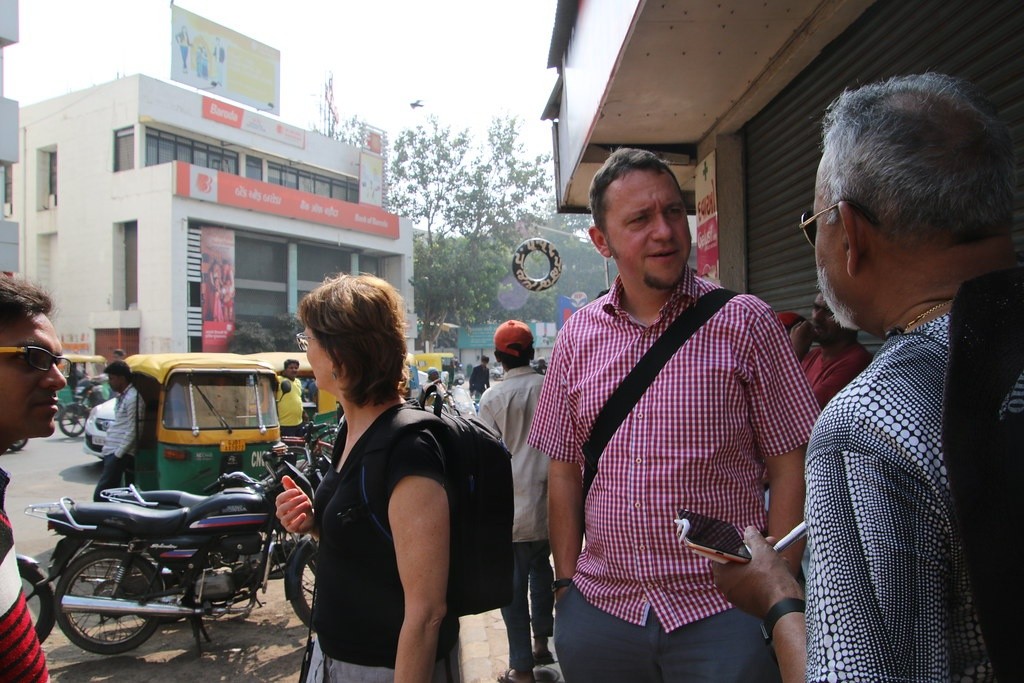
93,359,146,502
202,254,234,324
92,349,126,382
0,272,66,683
789,293,871,406
534,358,547,376
709,75,1016,683
423,368,444,404
469,356,489,403
277,359,309,437
526,149,825,683
275,275,461,683
480,320,554,683
70,364,90,404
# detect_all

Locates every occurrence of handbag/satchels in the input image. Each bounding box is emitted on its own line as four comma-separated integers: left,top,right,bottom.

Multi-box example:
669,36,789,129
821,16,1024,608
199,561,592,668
298,640,315,683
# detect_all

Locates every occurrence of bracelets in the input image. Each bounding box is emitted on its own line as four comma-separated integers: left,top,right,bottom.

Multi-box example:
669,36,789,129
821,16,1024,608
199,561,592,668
760,597,806,647
551,579,572,592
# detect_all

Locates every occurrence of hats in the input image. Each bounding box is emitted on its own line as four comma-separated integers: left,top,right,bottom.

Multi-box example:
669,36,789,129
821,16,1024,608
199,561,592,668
777,311,818,343
494,319,534,356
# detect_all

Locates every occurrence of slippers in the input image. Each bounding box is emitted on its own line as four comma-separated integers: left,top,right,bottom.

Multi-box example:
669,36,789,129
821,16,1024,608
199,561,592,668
497,668,536,683
532,650,556,666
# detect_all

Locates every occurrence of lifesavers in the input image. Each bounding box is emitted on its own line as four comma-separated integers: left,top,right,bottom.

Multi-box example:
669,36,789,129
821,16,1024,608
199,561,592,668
512,238,562,292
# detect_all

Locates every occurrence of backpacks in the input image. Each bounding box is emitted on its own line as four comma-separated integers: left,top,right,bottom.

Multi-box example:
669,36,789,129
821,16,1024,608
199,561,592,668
358,384,516,619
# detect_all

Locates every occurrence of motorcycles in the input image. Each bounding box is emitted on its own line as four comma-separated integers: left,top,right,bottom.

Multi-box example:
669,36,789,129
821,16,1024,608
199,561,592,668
15,422,340,656
54,351,465,496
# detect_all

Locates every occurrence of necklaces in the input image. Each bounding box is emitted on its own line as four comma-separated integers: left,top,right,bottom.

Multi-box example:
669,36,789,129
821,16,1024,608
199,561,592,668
904,300,951,330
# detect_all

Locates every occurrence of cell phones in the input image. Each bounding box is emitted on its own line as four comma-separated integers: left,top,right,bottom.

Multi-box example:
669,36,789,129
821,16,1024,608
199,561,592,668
676,510,751,564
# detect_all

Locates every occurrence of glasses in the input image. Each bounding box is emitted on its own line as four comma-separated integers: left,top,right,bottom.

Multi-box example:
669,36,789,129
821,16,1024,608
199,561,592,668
0,346,72,378
798,199,882,249
296,334,321,351
